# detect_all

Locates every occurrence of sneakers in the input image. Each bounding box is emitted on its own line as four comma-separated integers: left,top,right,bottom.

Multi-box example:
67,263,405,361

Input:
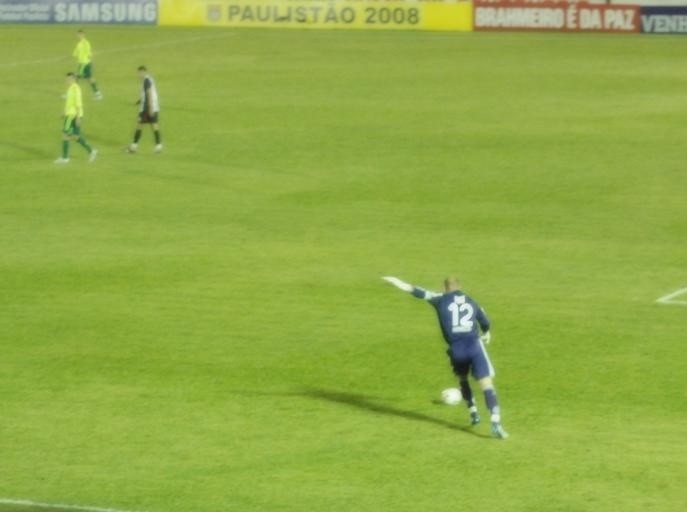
53,157,69,165
59,93,68,99
88,149,98,163
124,144,138,153
152,144,162,154
93,93,103,100
471,415,479,426
492,421,509,440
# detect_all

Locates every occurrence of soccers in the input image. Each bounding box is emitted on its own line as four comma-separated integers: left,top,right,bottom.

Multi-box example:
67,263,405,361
443,389,462,406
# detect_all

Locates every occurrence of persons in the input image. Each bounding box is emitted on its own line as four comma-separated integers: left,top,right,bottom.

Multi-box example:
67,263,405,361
60,28,103,100
381,273,509,439
52,71,99,163
120,64,164,154
